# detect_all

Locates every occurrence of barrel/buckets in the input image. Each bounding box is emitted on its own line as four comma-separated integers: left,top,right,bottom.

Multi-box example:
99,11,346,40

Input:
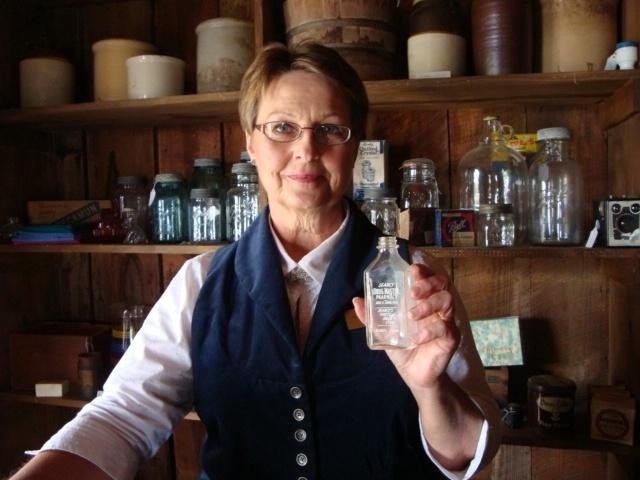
284,0,386,80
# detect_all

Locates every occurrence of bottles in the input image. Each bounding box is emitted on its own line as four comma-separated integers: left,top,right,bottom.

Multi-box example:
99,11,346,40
111,175,150,245
528,125,582,244
364,236,419,349
112,305,151,357
150,173,189,242
360,197,401,235
78,351,102,400
459,118,528,246
189,158,227,245
228,151,262,243
402,158,439,208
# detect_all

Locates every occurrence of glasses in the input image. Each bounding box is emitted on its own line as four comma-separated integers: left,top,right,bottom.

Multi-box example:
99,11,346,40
252,122,357,147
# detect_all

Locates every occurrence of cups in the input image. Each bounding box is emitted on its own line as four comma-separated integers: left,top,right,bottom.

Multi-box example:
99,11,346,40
19,56,74,108
405,30,466,80
91,36,158,101
193,18,254,93
125,54,186,101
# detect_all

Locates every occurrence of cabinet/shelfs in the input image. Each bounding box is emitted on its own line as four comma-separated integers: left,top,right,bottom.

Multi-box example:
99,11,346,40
3,5,640,475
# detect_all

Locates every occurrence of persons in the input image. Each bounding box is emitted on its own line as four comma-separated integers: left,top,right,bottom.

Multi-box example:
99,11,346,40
6,33,505,480
